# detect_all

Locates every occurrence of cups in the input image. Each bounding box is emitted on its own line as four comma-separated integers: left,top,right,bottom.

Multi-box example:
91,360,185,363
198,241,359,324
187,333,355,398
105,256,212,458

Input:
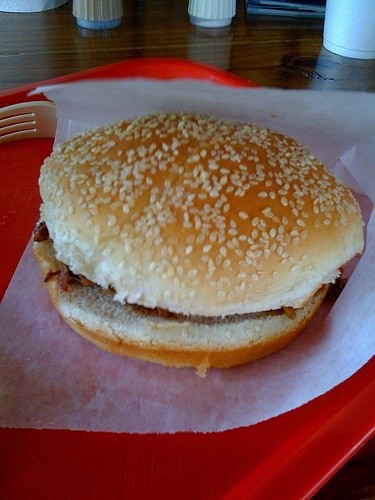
322,0,375,59
72,0,123,31
188,0,237,28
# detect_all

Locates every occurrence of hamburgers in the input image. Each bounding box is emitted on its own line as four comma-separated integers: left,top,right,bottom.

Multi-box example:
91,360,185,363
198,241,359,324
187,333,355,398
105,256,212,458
32,111,362,369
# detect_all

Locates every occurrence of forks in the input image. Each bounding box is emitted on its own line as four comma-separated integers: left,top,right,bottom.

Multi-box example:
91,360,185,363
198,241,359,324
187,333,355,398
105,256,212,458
0,101,58,144
